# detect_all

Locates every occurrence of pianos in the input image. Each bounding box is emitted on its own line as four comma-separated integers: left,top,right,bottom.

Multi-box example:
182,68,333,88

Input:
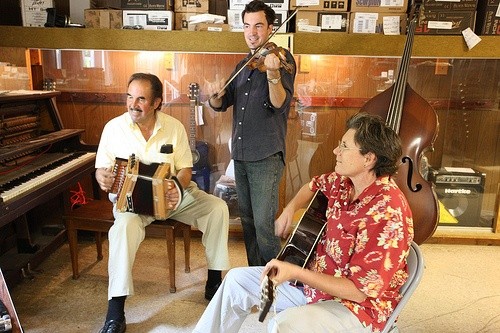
0,90,99,293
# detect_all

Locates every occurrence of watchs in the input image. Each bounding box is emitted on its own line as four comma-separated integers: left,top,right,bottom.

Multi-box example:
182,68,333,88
267,76,281,84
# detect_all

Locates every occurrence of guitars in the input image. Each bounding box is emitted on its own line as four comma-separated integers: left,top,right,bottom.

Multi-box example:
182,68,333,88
186,82,210,195
250,189,329,323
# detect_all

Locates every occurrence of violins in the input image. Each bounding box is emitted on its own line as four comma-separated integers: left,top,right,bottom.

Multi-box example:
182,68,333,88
244,42,296,74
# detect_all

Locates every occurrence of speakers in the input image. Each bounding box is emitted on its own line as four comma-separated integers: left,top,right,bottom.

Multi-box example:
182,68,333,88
428,167,486,228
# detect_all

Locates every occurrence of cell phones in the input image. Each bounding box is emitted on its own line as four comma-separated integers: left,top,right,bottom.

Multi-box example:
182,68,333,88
160,144,173,154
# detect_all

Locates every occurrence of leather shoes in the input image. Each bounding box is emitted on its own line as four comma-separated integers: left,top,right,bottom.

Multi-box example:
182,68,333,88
204,280,222,300
99,312,126,333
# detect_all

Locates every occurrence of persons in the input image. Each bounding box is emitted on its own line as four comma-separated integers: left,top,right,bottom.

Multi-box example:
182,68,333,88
207,1,297,267
94,72,229,333
190,111,414,333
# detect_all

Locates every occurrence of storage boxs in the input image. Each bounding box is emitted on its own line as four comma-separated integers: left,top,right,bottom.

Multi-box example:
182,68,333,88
295,11,350,34
349,13,407,35
410,0,500,35
290,0,348,11
350,0,409,13
84,0,289,35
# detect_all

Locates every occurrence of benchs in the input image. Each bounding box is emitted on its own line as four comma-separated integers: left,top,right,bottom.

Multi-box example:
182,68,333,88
63,200,191,293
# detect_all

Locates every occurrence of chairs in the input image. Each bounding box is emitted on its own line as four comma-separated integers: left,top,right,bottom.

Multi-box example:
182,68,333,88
381,241,425,333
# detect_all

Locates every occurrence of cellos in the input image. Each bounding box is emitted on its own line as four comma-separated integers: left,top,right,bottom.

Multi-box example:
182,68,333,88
359,2,440,246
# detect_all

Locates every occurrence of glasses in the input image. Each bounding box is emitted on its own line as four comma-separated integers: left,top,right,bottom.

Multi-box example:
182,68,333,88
337,140,363,153
